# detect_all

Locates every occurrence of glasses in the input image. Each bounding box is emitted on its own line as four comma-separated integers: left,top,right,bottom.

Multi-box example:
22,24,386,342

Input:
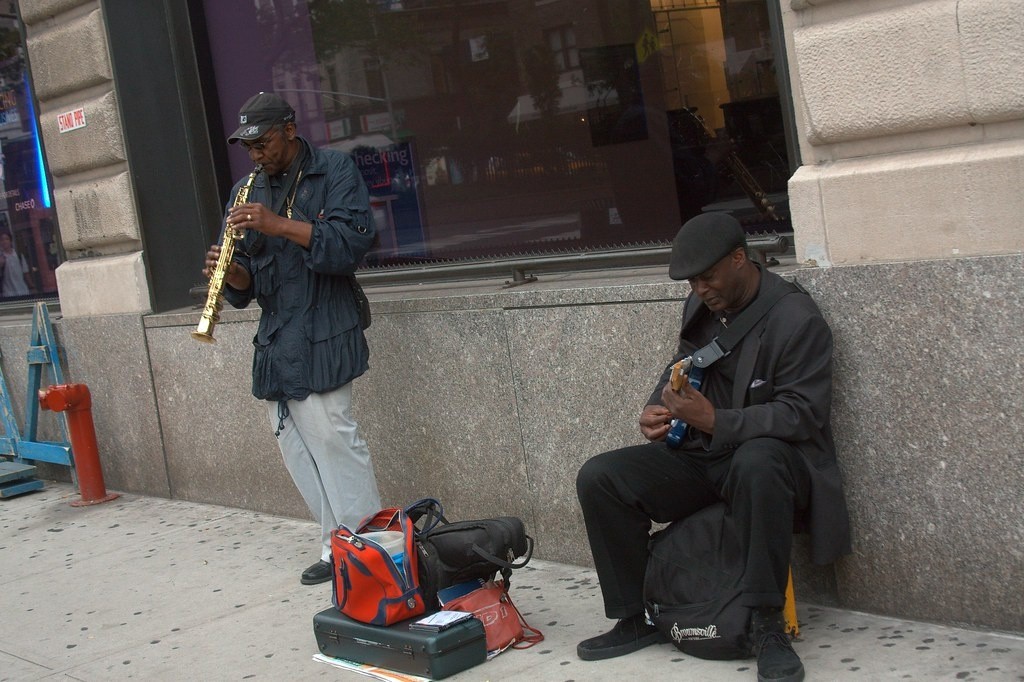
240,128,281,152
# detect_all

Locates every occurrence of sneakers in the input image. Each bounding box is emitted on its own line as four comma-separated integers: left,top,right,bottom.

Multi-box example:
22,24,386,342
748,608,804,682
578,613,663,661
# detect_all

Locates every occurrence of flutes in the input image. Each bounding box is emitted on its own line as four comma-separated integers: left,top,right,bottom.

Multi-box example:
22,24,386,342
187,162,264,346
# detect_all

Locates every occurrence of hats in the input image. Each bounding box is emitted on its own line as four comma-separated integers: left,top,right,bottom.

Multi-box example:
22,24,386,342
227,92,295,145
669,211,746,281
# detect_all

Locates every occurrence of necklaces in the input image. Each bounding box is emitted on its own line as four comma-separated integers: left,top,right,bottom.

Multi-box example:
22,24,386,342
285,169,303,220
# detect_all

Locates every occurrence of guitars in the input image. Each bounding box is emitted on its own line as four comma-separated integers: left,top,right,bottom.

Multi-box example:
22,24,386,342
663,356,705,447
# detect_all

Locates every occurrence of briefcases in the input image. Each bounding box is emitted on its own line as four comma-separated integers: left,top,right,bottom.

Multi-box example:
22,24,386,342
313,596,487,680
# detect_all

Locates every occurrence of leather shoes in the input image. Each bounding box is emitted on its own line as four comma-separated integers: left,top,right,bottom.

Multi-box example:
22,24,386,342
301,557,333,585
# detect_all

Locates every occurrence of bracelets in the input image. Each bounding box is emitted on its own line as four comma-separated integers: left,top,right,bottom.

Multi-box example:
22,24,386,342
28,287,38,291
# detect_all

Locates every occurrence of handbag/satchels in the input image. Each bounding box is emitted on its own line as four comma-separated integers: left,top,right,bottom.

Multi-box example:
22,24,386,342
443,577,545,649
412,514,536,592
328,507,425,625
346,270,371,331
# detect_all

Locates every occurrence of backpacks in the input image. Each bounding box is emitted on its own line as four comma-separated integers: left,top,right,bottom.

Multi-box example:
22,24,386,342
642,501,758,661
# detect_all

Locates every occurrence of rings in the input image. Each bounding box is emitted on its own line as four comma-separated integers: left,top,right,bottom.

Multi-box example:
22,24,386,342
246,213,253,221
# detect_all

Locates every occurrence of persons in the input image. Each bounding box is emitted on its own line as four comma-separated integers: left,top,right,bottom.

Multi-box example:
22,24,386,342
575,212,850,682
201,91,383,584
0,229,39,299
